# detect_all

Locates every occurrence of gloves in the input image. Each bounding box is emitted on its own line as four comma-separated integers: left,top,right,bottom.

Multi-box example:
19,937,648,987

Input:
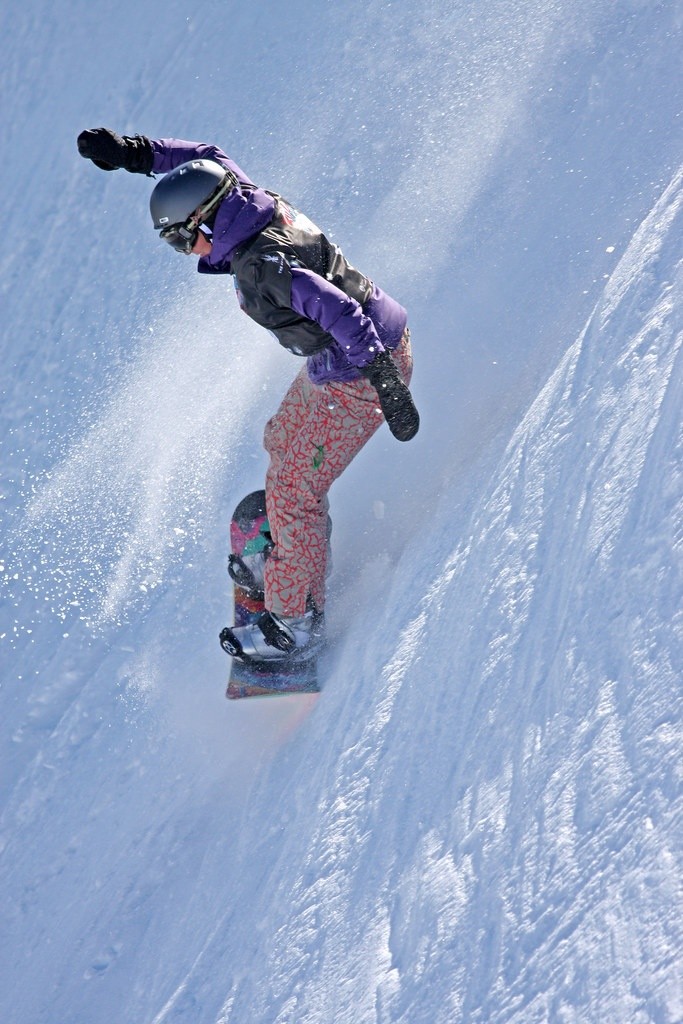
362,347,420,442
77,127,157,180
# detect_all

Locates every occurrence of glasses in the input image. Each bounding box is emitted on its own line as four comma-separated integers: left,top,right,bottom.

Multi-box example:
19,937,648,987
159,225,200,254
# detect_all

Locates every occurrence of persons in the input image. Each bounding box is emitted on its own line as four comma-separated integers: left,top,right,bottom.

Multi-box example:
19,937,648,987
78,127,420,672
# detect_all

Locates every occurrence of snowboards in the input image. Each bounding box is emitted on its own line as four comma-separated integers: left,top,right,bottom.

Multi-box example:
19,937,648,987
222,488,333,698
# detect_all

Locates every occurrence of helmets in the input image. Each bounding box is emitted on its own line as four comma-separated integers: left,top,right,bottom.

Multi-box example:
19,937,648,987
150,159,238,229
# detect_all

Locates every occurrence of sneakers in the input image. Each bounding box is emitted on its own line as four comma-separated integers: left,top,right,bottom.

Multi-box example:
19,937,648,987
220,610,325,663
229,532,274,590
